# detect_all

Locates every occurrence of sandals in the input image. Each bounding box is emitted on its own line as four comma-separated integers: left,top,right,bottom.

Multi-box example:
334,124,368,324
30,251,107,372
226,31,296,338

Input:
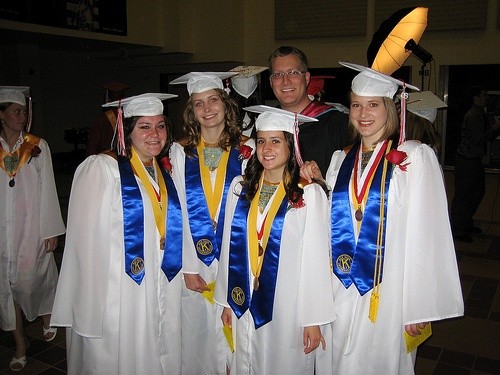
10,354,27,372
43,324,58,342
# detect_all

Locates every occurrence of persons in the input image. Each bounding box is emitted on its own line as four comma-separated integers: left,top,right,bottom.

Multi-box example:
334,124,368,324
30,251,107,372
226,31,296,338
170,70,256,375
47,92,182,375
208,105,336,375
60,113,119,179
452,86,500,243
296,60,464,375
0,85,65,371
269,46,352,178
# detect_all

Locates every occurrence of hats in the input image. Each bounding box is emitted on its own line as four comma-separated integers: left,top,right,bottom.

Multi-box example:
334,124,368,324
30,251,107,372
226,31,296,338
242,105,319,167
0,86,34,131
396,90,449,123
101,92,179,156
225,65,270,99
101,81,132,101
338,60,420,146
169,71,239,94
307,75,336,95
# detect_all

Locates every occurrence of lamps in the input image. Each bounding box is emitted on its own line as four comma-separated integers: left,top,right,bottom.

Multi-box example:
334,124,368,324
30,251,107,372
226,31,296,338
405,40,433,64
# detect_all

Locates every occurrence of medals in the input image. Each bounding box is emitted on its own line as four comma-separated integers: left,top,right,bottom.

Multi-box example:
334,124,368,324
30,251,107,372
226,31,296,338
159,237,166,251
9,180,15,187
355,210,363,221
213,220,217,231
258,246,263,256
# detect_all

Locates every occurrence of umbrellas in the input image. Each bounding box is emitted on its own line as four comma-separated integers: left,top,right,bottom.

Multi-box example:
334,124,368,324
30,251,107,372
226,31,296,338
366,6,428,78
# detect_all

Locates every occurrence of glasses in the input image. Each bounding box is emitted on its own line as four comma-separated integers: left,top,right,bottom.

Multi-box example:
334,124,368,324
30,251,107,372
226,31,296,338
272,70,303,80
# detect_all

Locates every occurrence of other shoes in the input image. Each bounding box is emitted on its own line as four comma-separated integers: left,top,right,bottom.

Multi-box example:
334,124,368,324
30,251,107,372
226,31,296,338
451,224,484,242
